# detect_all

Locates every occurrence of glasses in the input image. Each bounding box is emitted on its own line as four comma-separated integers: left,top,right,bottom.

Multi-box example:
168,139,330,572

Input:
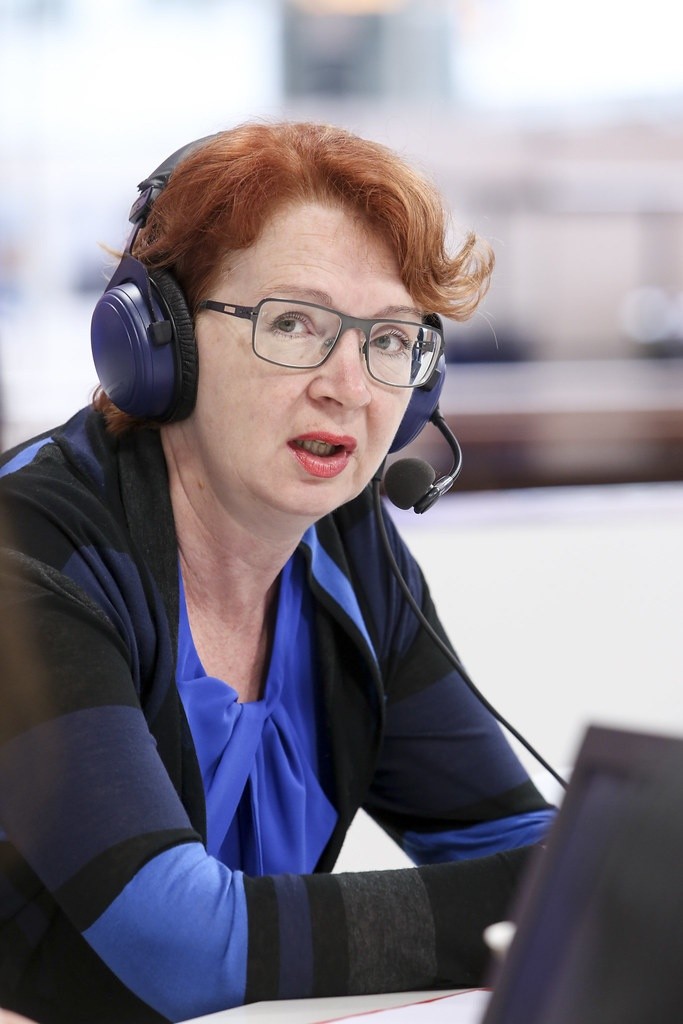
199,297,445,388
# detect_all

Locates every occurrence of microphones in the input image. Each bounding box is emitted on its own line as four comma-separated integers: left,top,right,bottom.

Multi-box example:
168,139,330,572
383,417,462,513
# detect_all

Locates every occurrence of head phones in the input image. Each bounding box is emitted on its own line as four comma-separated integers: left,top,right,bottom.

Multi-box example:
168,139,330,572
90,129,446,454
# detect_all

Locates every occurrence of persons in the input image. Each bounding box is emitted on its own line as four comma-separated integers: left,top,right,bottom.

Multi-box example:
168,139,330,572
0,124,559,1023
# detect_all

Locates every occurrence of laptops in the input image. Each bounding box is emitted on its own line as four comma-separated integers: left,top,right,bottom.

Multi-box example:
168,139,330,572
483,724,682,1024
